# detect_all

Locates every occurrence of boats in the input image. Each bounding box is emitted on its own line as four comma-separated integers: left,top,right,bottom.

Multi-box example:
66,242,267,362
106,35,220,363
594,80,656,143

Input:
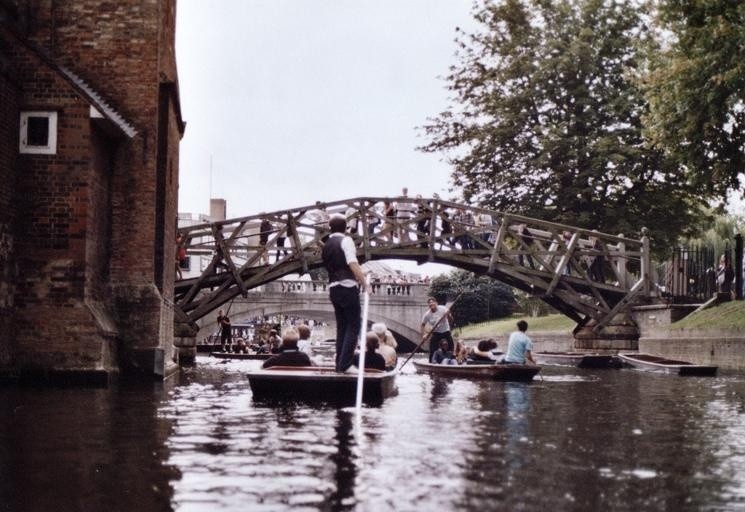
205,345,282,361
244,338,398,404
195,322,255,351
412,357,541,384
613,351,718,380
532,349,618,367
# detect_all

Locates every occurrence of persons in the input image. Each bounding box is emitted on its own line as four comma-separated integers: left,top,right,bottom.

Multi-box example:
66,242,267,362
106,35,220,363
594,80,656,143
268,329,284,352
177,232,185,280
309,271,328,291
439,208,499,260
346,208,362,248
456,338,505,364
368,270,431,295
307,202,329,254
276,214,289,262
503,320,536,365
263,327,310,368
562,231,579,274
716,255,734,298
368,188,430,249
321,212,368,373
217,310,233,353
234,338,248,353
588,229,604,282
354,323,396,371
296,325,314,360
259,212,271,267
422,297,454,363
256,341,269,354
515,221,536,270
432,338,458,365
